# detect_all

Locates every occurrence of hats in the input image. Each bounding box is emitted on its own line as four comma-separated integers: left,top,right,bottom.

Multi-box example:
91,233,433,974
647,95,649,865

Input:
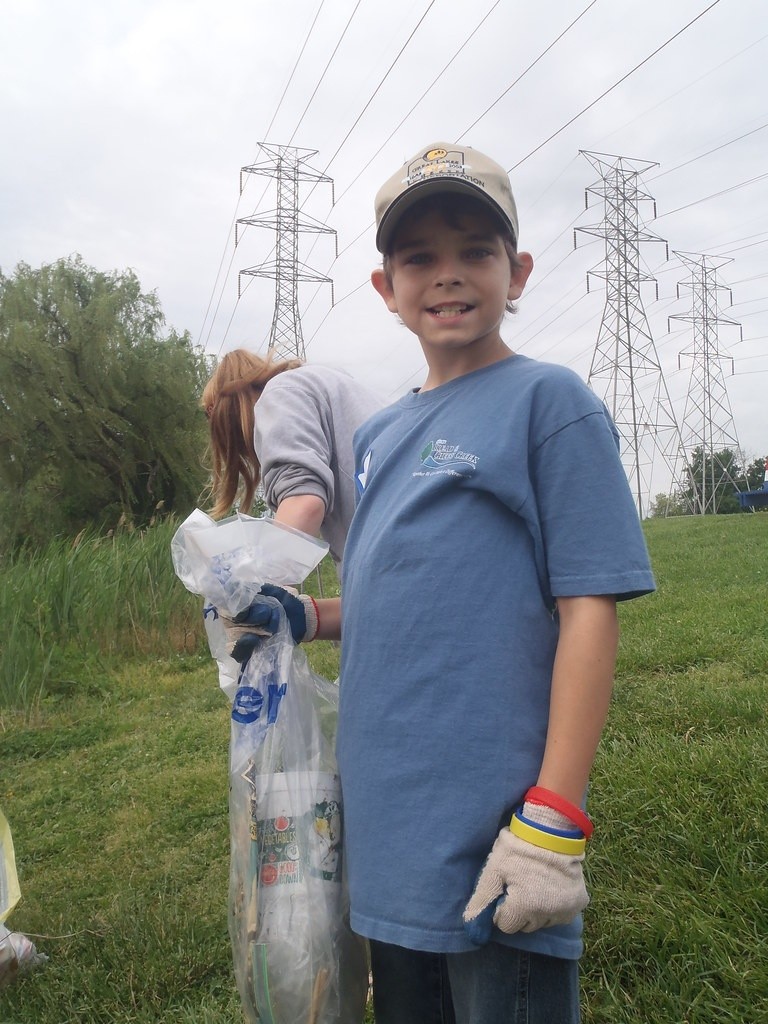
375,142,519,251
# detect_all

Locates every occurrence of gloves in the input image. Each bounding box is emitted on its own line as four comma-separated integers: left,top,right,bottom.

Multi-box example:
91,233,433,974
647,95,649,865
462,799,590,935
220,583,319,654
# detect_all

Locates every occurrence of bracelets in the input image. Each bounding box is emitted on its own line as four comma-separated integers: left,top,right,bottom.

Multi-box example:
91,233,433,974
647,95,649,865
509,786,594,856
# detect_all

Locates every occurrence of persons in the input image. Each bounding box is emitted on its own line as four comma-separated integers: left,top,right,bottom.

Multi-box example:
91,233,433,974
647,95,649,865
196,346,389,589
228,143,663,1024
